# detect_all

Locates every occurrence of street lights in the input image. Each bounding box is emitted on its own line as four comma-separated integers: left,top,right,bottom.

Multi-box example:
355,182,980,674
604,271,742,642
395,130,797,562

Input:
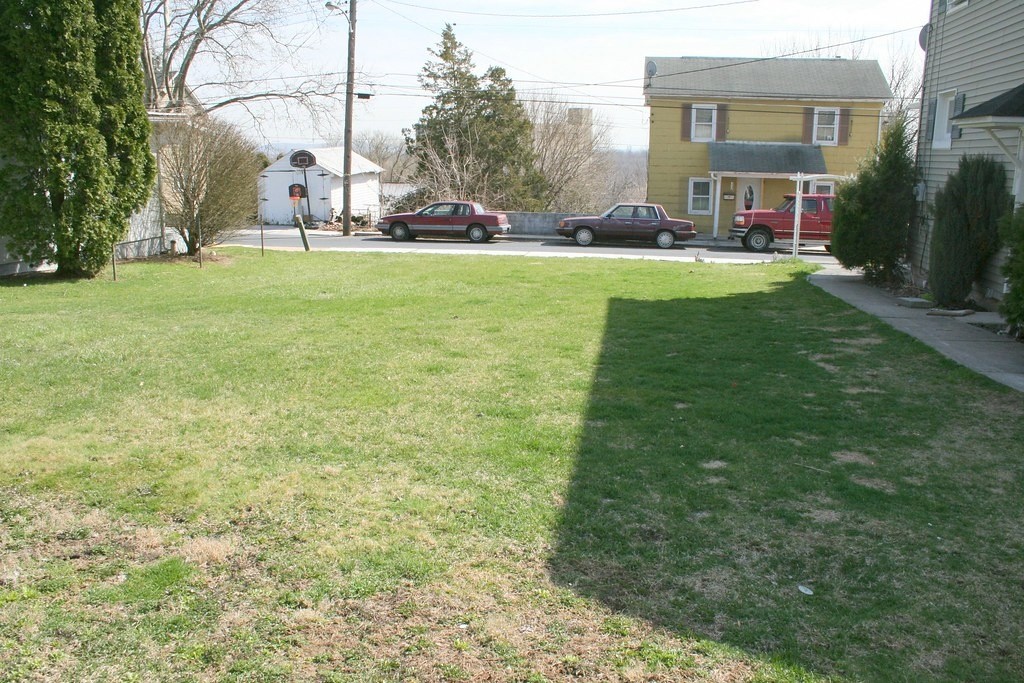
325,0,355,236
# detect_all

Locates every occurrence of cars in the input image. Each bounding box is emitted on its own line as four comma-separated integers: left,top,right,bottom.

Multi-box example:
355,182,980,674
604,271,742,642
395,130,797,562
555,202,698,249
377,200,512,243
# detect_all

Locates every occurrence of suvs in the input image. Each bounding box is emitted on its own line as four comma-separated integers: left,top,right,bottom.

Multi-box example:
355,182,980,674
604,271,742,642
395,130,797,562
728,192,850,254
